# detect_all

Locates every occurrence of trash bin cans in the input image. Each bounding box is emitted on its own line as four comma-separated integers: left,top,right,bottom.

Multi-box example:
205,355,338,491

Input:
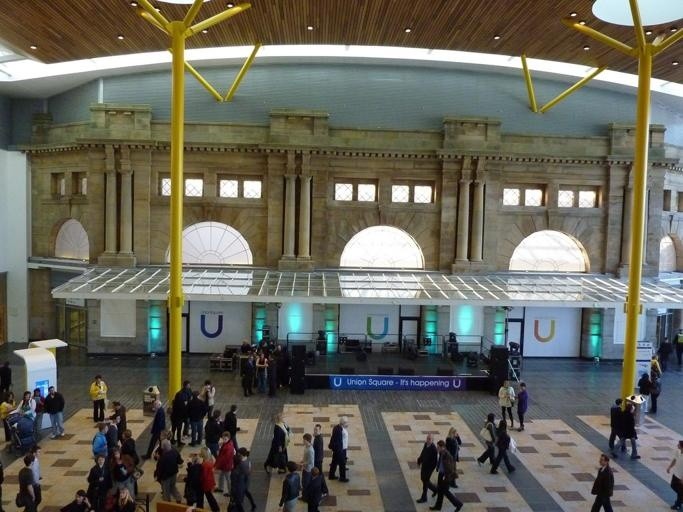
625,395,647,426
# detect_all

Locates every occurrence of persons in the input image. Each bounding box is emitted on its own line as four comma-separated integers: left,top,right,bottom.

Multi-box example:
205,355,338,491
19,446,42,510
667,440,682,511
144,380,257,510
2,386,65,445
71,400,144,510
411,378,528,511
636,324,683,411
589,453,615,512
608,398,627,455
615,404,641,459
91,375,107,422
265,413,351,510
241,339,304,397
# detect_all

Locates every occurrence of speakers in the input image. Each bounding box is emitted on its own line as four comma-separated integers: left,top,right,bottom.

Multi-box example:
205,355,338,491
339,365,356,375
437,366,454,376
288,344,306,395
377,366,393,374
398,360,415,374
489,344,509,396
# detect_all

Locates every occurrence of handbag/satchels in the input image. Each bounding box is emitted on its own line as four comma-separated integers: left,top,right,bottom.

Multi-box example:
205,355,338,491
16,493,24,508
320,478,328,494
133,469,144,480
481,429,492,442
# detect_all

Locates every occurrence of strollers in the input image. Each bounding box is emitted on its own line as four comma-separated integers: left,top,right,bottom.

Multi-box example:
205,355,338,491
6,409,43,457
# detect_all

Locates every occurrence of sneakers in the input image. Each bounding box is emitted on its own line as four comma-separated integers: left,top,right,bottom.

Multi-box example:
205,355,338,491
213,487,229,497
631,455,642,459
671,504,683,510
478,459,481,466
143,455,151,459
51,433,64,438
510,424,522,432
263,463,285,474
250,504,256,511
489,467,515,475
330,475,349,483
415,490,463,512
170,436,200,446
